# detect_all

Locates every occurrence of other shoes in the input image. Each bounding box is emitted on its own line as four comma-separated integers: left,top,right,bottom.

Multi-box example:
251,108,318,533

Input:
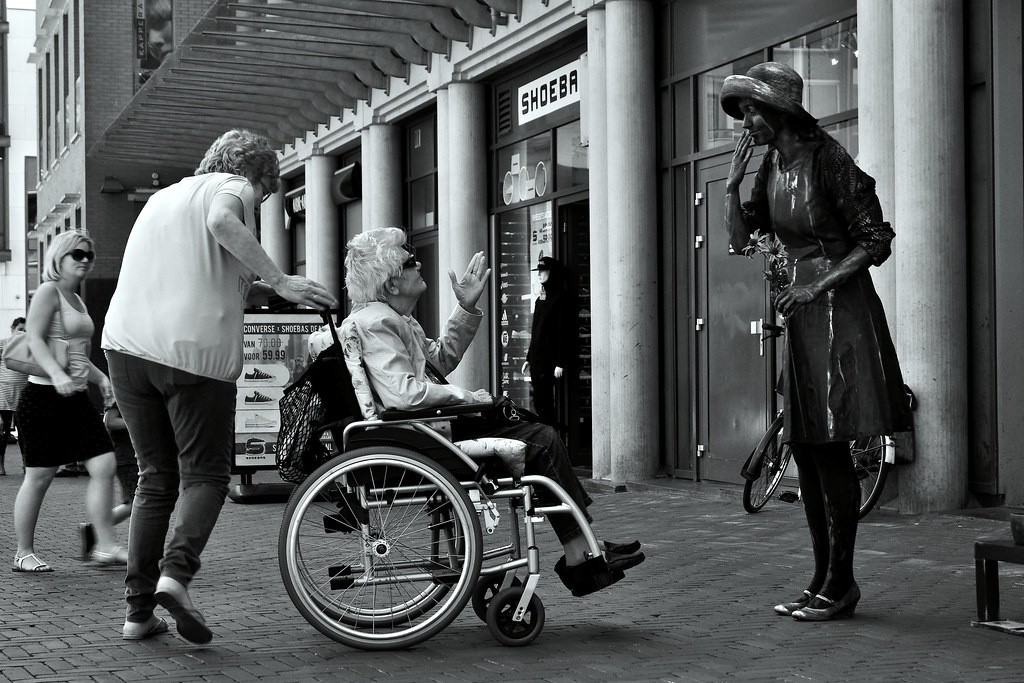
565,540,645,580
0,464,6,475
54,469,78,478
512,330,531,339
579,287,591,380
513,372,524,380
80,523,96,561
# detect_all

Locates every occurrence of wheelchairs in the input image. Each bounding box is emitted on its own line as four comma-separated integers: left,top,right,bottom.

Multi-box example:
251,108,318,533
250,278,626,655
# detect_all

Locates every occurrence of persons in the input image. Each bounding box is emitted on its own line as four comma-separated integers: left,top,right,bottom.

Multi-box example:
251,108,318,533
520,257,582,452
345,228,647,588
100,127,339,644
0,227,143,573
718,63,913,621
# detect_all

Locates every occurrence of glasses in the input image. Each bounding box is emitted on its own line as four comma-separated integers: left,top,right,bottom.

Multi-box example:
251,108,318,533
403,254,417,269
260,181,271,202
65,249,95,262
18,328,26,332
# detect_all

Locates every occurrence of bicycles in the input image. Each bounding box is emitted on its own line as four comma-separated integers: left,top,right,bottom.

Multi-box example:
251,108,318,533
740,322,898,520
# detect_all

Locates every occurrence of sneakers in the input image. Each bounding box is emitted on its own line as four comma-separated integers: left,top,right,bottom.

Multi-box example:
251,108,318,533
245,368,277,427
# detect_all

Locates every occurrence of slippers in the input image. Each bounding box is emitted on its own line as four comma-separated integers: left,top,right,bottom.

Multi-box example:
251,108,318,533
154,588,212,644
122,617,169,639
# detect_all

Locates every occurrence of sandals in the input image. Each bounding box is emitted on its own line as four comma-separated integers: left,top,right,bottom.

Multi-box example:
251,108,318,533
12,553,55,572
92,546,127,571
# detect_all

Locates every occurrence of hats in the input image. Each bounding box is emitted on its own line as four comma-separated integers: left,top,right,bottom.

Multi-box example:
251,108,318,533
529,256,554,271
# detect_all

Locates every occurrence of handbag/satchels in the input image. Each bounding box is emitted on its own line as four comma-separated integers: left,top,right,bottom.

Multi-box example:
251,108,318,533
3,282,69,377
481,394,538,423
